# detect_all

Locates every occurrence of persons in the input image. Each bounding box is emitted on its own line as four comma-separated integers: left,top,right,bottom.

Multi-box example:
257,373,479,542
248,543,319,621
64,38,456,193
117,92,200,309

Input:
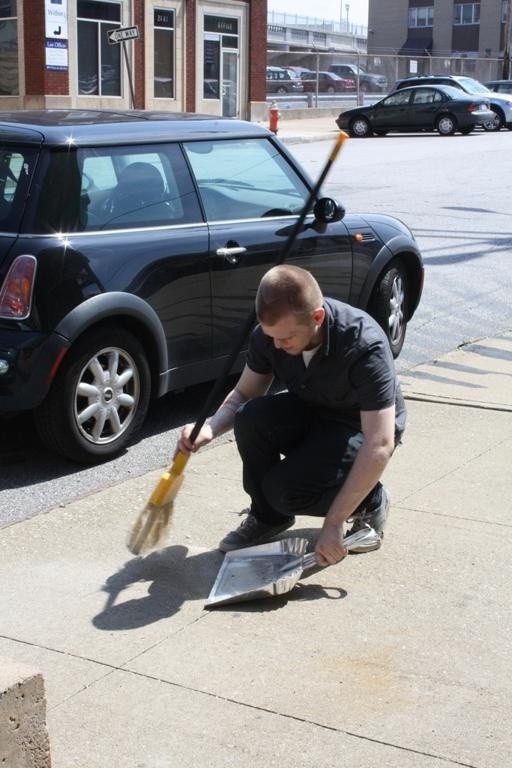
170,259,411,570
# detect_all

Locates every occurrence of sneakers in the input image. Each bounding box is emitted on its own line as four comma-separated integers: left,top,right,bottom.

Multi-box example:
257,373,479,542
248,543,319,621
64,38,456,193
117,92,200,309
344,489,390,554
218,509,298,554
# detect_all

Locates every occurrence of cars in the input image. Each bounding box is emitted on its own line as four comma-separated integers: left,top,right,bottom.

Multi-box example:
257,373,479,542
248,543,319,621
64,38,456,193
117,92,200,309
204,78,236,99
0,108,424,464
266,63,512,137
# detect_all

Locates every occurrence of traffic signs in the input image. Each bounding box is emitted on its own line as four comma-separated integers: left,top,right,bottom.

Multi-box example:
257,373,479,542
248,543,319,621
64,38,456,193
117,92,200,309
106,25,140,45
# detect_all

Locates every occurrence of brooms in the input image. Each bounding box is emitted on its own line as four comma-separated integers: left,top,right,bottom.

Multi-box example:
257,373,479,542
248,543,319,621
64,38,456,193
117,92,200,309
129,130,349,560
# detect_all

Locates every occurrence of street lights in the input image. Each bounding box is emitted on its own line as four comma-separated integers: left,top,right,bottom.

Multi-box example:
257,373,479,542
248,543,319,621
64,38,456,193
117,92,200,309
345,3,350,33
